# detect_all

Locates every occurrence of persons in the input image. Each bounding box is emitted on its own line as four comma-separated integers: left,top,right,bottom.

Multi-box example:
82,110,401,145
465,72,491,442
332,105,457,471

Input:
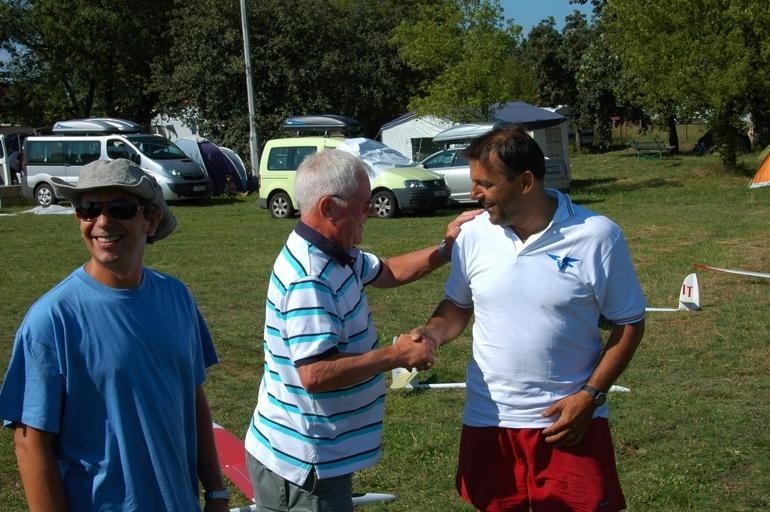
8,143,21,185
16,152,24,183
244,151,484,512
408,129,648,512
0,159,238,512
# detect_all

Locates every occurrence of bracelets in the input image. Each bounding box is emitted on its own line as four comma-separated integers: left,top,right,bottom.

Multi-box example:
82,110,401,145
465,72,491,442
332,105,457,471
204,488,230,501
438,239,451,263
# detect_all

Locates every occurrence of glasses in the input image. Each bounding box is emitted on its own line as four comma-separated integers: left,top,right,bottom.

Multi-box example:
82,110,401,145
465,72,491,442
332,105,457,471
330,194,375,216
77,200,145,220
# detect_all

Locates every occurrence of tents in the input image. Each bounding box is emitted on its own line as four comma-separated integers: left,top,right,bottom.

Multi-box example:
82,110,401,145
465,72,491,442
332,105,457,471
170,134,247,197
748,144,770,188
379,110,463,164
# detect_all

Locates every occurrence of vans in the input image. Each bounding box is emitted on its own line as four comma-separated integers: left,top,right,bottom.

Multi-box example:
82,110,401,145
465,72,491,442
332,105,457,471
21,117,213,204
257,116,448,217
418,102,573,205
0,124,44,186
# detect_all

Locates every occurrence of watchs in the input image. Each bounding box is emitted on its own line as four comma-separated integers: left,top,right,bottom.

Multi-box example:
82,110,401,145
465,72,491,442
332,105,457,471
581,383,607,406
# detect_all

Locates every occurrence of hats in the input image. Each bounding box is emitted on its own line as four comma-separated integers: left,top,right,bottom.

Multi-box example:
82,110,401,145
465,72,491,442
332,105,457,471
51,158,176,244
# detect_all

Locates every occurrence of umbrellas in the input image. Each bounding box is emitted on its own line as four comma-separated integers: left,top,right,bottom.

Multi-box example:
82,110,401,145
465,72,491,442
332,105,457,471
473,101,566,132
